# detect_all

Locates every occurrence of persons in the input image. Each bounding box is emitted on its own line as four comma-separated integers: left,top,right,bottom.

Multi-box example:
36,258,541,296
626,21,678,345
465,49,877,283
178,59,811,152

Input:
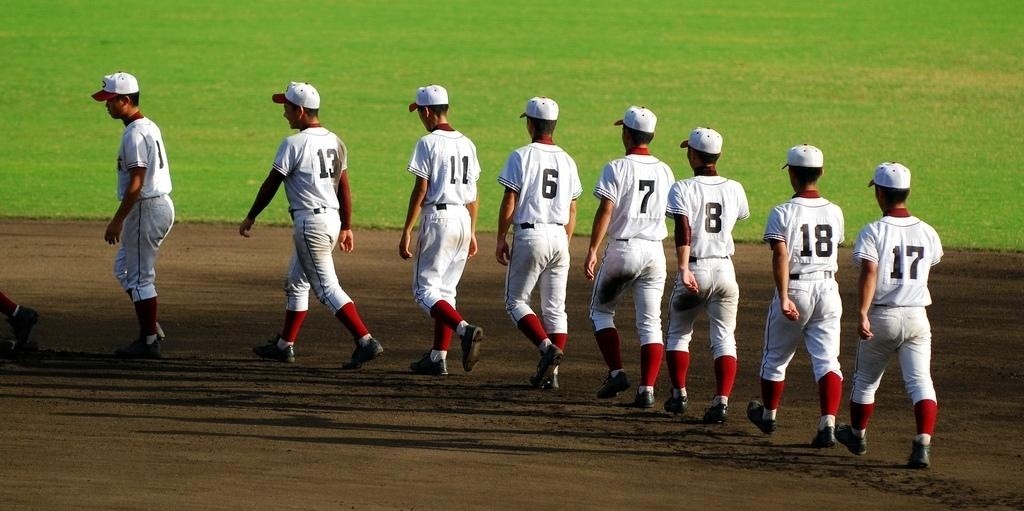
495,95,583,391
582,105,677,410
663,127,751,426
89,72,175,360
832,162,944,471
747,143,844,450
397,83,484,377
237,80,384,372
0,290,40,359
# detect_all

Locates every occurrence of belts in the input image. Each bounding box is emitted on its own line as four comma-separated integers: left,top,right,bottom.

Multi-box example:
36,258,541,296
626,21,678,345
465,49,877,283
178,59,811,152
521,222,533,229
790,274,799,281
314,208,324,213
436,203,445,209
617,238,628,241
688,256,697,263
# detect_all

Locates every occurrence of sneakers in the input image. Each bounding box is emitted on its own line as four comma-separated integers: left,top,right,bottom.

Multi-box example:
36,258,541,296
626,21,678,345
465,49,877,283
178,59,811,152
703,402,727,422
597,371,629,400
252,342,295,364
460,323,482,373
531,344,563,388
115,323,165,359
664,397,689,414
746,400,777,433
811,424,866,455
410,354,447,376
633,386,654,407
7,305,37,354
344,337,381,370
907,441,932,467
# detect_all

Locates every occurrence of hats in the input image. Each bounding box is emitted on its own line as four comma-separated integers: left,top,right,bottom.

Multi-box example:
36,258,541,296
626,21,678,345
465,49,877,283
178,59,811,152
782,144,823,169
520,96,558,122
868,162,911,190
408,86,448,112
680,127,723,154
272,82,320,109
91,72,139,101
614,108,658,133
